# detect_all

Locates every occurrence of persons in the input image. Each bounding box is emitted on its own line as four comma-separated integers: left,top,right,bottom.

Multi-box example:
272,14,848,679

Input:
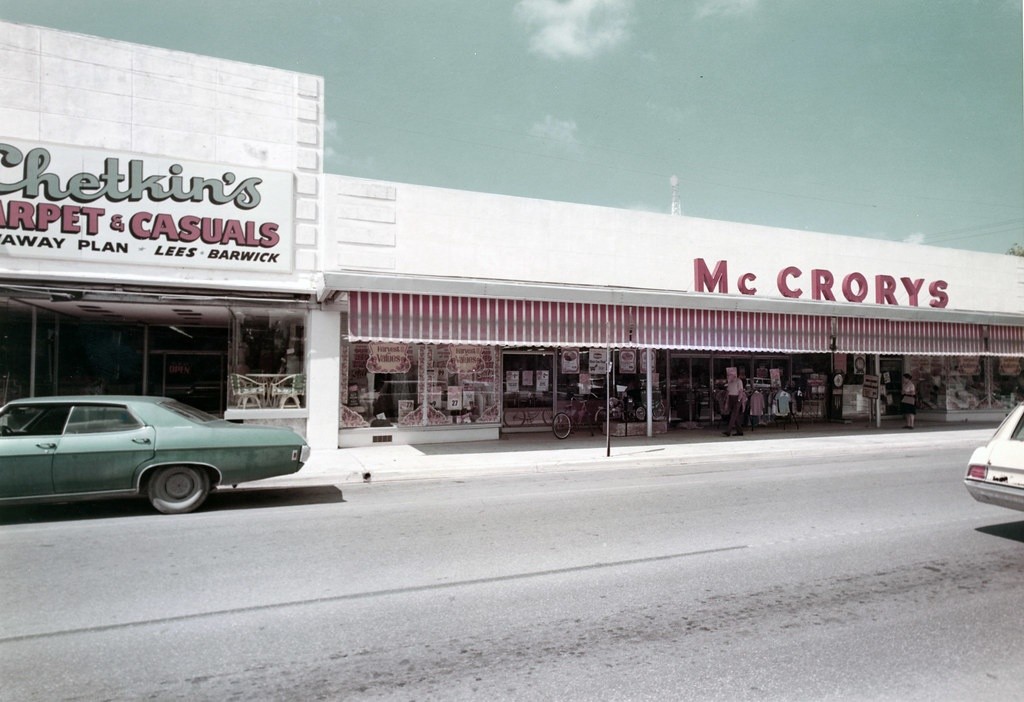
719,370,744,437
901,373,916,429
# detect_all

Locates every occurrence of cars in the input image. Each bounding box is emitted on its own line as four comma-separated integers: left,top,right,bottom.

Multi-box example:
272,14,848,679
0,392,313,515
964,402,1023,514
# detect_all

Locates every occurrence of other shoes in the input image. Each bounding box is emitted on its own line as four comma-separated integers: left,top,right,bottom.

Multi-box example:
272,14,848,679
902,426,913,429
720,432,731,437
732,432,743,436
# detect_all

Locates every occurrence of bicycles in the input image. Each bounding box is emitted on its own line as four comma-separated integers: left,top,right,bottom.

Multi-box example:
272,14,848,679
594,398,625,432
503,390,554,428
551,395,595,439
